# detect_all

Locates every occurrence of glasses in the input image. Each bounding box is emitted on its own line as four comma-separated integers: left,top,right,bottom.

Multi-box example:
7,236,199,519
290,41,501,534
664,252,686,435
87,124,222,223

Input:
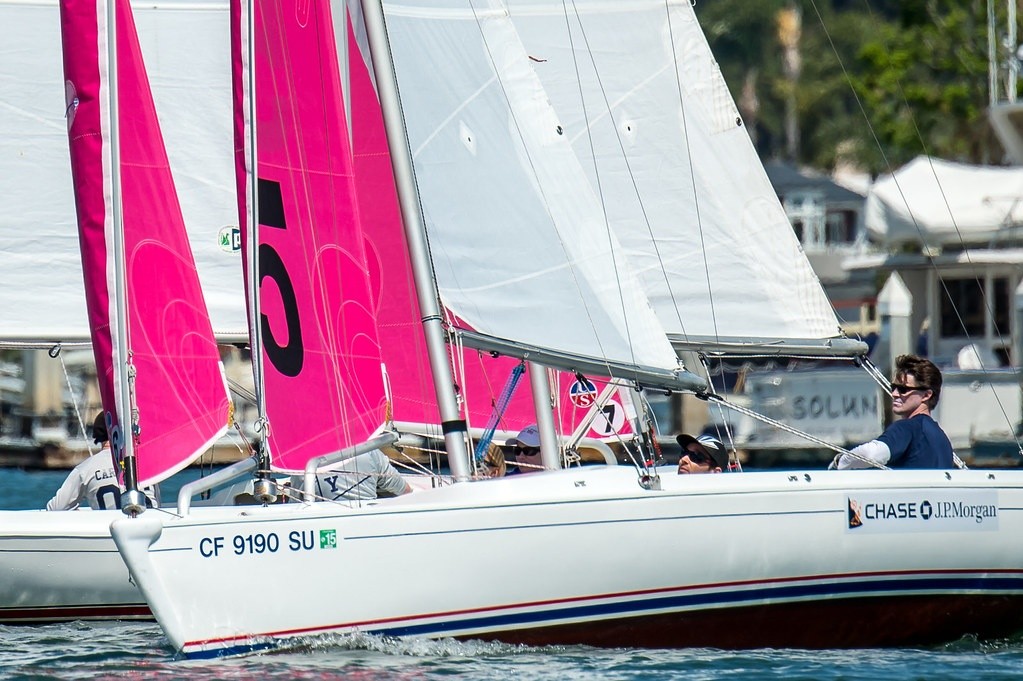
891,383,930,394
514,446,541,456
680,449,714,464
471,459,498,469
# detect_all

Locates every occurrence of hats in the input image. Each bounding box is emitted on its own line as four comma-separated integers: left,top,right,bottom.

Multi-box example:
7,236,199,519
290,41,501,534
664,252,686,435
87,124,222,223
677,434,729,473
468,438,506,478
505,425,541,447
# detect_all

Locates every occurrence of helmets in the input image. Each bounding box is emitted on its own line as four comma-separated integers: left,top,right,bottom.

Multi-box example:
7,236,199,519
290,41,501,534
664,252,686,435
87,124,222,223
92,409,108,444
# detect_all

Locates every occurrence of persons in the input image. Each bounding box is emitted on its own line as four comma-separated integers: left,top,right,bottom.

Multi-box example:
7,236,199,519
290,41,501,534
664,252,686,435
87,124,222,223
285,443,412,504
465,422,542,480
828,355,955,471
45,411,162,511
676,432,729,474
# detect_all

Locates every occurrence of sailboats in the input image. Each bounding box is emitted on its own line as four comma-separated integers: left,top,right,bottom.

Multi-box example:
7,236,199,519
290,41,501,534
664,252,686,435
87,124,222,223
0,1,1021,665
